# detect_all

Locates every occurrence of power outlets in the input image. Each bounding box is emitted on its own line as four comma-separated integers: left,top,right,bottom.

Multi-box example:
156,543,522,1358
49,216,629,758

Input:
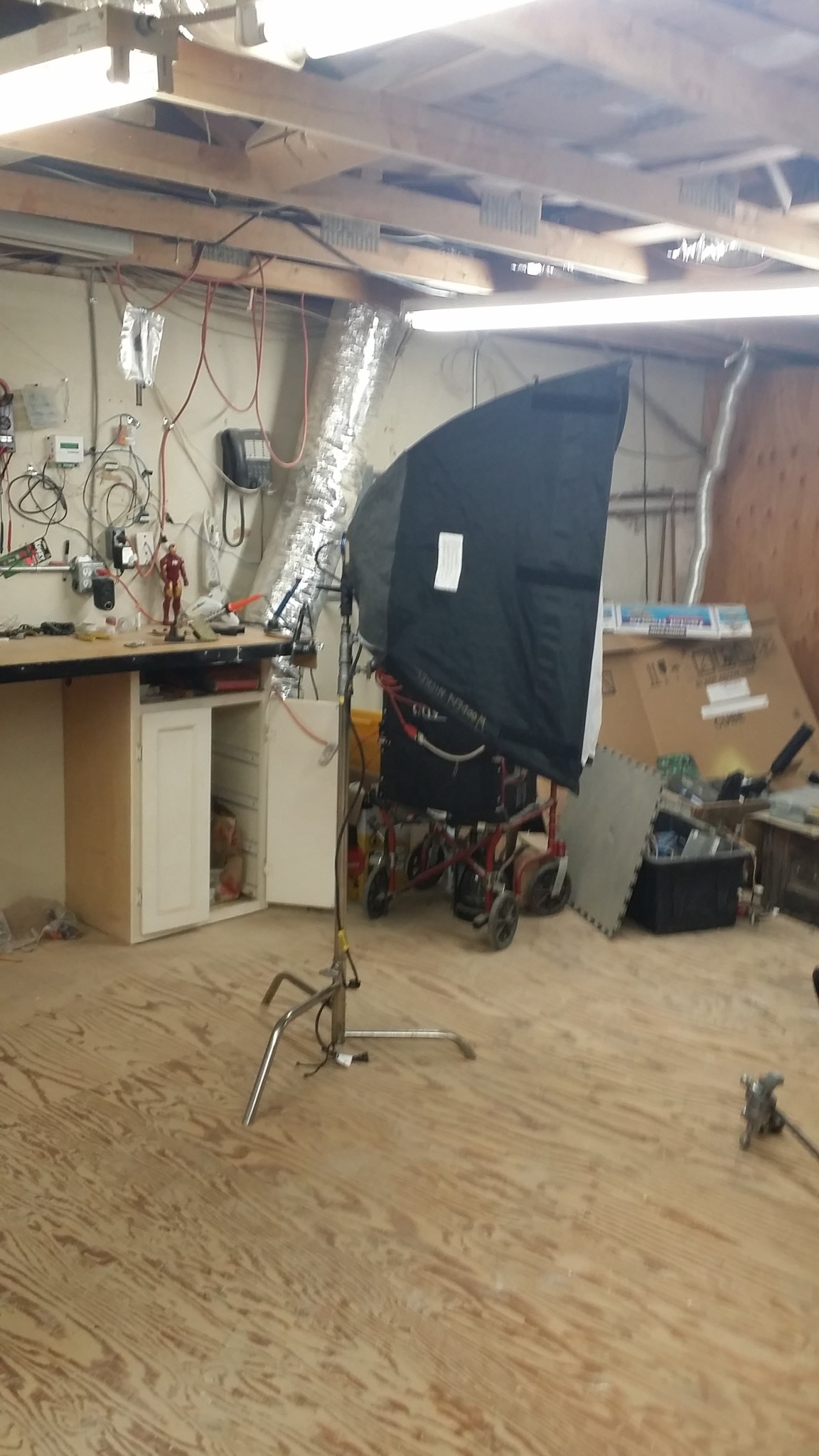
70,559,108,595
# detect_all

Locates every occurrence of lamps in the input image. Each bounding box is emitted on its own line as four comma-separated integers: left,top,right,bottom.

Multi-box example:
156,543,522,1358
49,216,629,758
233,0,539,74
394,267,819,337
0,3,175,138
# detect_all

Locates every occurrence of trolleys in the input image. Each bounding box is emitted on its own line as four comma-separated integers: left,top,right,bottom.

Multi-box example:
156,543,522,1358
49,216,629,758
358,661,575,952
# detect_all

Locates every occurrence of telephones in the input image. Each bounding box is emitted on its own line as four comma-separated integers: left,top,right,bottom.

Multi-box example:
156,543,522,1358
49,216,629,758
223,428,271,489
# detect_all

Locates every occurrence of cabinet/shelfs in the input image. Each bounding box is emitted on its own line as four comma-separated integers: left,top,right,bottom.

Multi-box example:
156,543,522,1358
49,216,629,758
134,694,338,935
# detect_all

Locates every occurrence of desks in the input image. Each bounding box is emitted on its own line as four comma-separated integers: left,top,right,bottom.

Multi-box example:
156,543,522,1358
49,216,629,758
0,619,291,947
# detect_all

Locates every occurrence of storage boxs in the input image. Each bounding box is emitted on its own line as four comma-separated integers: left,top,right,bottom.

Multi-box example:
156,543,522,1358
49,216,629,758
741,810,819,923
627,807,760,938
602,600,754,643
597,622,819,792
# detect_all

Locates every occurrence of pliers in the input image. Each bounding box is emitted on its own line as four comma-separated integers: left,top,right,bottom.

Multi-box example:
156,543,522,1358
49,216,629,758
74,632,109,641
